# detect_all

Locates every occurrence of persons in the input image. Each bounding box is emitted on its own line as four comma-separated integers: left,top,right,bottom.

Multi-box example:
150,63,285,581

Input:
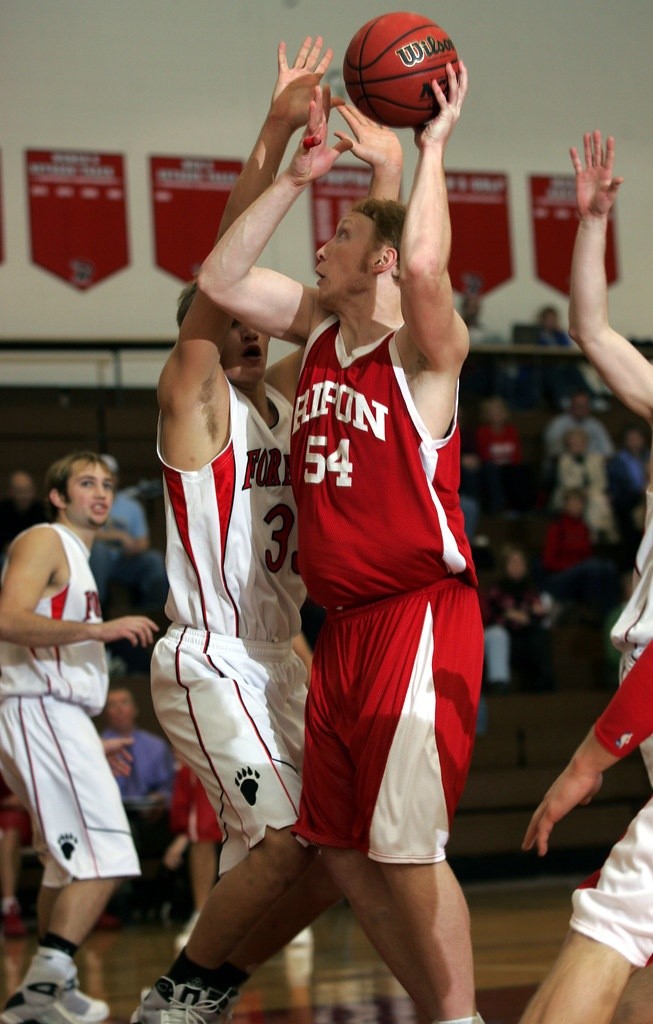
0,35,653,1024
0,454,158,1024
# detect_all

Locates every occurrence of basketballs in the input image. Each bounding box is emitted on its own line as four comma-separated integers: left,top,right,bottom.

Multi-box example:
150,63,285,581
344,10,466,125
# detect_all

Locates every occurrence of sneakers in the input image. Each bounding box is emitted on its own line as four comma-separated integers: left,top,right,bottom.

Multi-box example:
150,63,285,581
129,975,238,1024
0,954,110,1024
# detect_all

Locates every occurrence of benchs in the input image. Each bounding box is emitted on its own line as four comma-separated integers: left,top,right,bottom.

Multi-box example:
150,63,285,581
429,603,649,858
